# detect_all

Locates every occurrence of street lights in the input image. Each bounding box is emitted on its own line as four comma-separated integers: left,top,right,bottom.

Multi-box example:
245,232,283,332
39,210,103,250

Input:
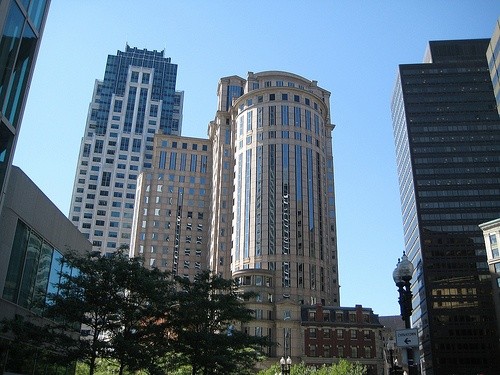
394,251,414,375
387,337,393,375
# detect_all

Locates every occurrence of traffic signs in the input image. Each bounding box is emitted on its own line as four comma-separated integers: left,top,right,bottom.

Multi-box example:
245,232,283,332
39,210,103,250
395,328,420,350
